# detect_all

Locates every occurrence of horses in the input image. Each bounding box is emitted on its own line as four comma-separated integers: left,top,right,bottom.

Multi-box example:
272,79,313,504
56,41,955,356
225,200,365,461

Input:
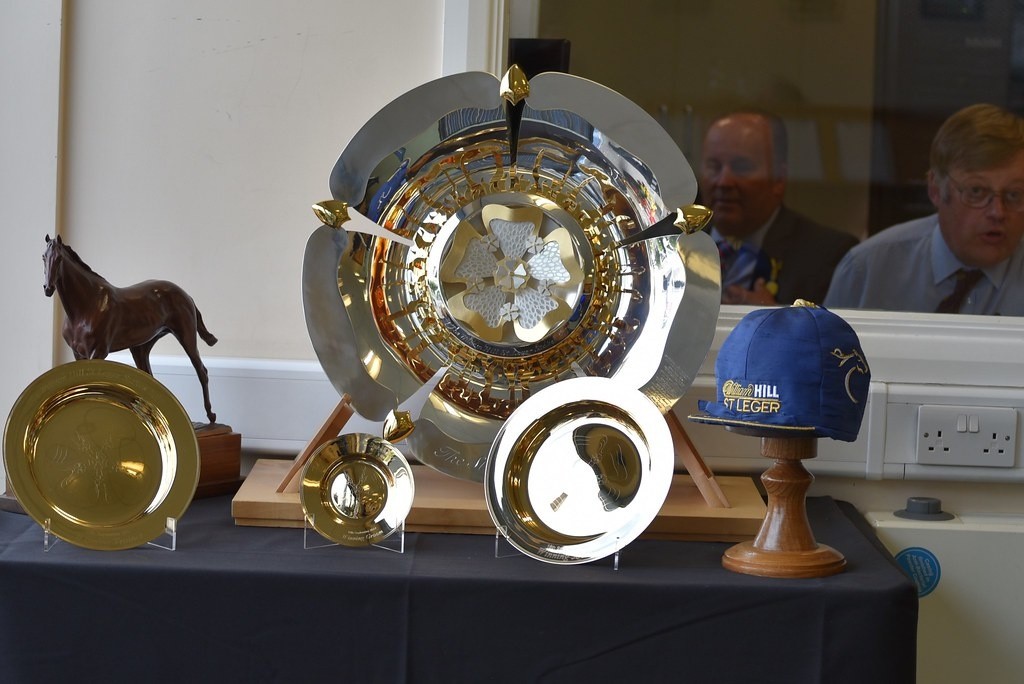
42,234,218,422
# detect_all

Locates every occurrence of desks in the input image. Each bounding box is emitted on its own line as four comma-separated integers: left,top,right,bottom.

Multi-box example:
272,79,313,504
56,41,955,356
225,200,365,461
0,472,924,684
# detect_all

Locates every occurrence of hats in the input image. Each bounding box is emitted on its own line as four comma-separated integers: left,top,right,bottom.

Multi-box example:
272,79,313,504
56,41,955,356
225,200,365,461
893,496,955,521
685,299,869,440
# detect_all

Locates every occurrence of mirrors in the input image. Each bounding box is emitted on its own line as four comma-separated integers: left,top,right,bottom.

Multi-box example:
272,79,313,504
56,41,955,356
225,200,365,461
500,1,1023,318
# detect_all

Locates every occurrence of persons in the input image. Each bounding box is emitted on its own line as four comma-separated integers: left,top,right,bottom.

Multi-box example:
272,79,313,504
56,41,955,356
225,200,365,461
823,103,1024,317
694,110,859,305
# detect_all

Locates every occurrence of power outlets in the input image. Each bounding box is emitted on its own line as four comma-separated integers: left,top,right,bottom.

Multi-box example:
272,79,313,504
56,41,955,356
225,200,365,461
916,405,1018,467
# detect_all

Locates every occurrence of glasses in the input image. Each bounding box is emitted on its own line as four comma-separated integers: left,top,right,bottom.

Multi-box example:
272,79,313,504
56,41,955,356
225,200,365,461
945,172,1024,212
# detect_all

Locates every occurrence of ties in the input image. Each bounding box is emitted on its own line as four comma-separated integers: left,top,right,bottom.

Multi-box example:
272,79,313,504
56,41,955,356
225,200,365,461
720,242,738,275
933,269,984,313
